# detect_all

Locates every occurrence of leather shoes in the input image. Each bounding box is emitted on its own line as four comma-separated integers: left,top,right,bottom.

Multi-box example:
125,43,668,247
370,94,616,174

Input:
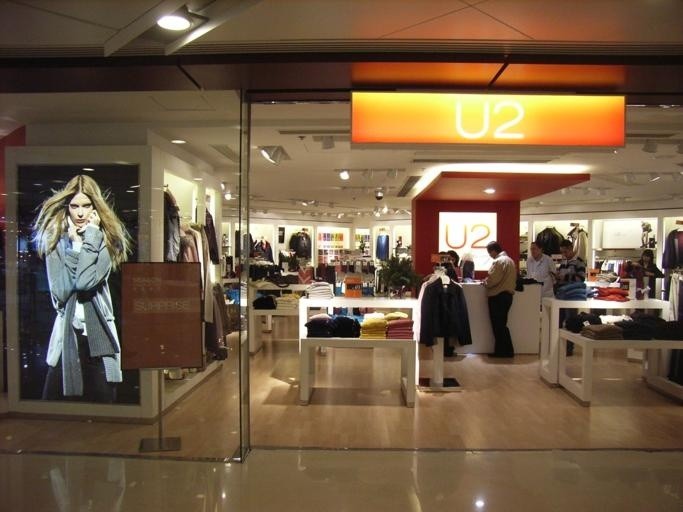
486,351,506,357
508,351,514,357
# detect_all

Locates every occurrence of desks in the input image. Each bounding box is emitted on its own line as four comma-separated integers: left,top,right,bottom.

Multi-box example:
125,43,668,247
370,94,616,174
539,298,683,403
247,276,419,409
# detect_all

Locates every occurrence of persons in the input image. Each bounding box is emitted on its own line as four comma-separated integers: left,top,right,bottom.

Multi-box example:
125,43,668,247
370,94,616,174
478,241,517,357
547,239,587,356
394,235,402,256
524,241,558,309
446,249,463,282
26,174,138,401
637,248,665,299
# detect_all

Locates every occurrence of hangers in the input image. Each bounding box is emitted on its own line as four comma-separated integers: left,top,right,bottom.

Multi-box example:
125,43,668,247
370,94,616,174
418,255,463,291
666,222,683,237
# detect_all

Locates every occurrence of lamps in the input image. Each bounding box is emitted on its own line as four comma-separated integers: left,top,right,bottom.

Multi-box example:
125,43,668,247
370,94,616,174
260,146,291,166
374,187,384,200
